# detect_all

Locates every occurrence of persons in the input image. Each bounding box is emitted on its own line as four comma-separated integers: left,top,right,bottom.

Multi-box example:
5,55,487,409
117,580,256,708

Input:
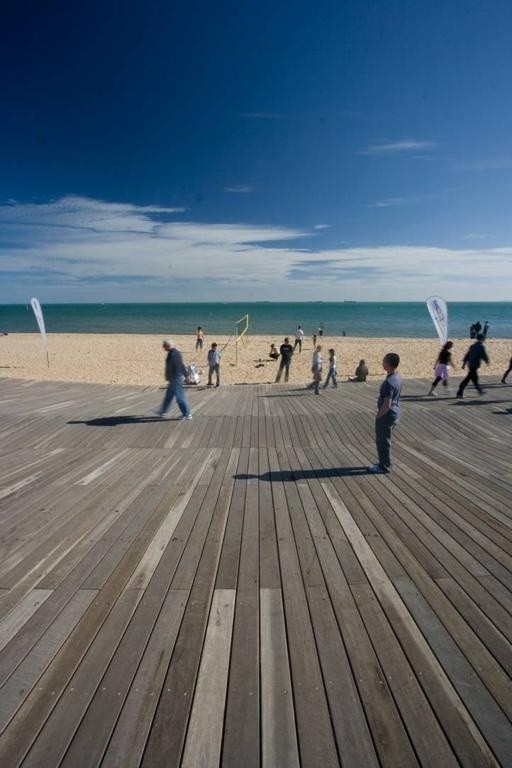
502,357,512,384
428,341,458,396
367,353,402,473
457,334,491,398
153,340,192,420
269,323,369,395
470,321,489,339
207,343,221,387
196,327,204,351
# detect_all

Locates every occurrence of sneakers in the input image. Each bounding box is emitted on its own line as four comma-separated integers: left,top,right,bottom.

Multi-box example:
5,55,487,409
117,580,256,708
428,391,437,395
454,394,465,399
478,390,488,396
368,460,389,473
446,388,454,393
305,386,340,395
178,413,192,419
